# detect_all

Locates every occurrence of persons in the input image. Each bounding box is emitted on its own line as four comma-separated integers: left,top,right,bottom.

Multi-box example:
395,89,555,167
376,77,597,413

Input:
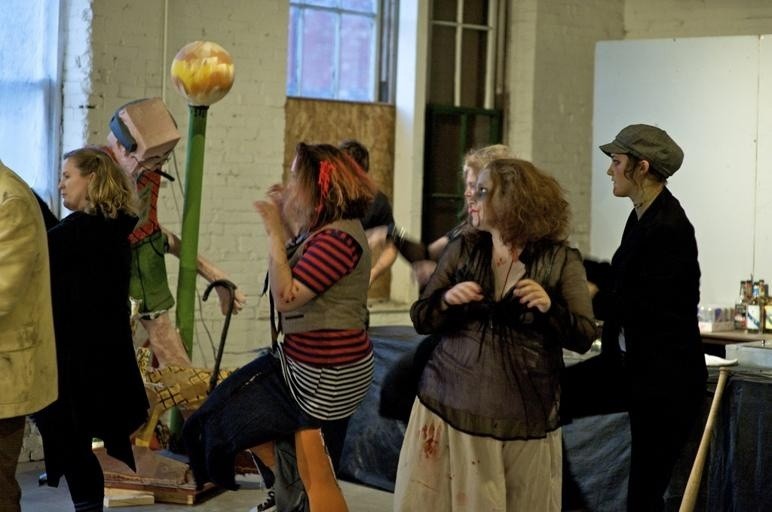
387,156,599,510
249,438,285,511
185,140,379,511
365,142,518,297
30,146,152,512
335,141,398,290
560,125,712,510
1,160,61,512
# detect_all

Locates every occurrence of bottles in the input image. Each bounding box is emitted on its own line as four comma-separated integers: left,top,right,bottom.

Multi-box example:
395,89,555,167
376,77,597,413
733,280,772,334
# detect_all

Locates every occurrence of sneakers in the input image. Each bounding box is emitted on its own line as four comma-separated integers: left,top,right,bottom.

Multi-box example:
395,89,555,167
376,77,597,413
250,489,277,512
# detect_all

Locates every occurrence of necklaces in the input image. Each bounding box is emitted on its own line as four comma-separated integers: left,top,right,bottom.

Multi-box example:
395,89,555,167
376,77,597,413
488,251,522,268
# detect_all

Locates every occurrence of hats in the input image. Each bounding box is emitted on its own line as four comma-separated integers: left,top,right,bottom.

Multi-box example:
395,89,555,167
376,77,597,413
598,124,684,178
